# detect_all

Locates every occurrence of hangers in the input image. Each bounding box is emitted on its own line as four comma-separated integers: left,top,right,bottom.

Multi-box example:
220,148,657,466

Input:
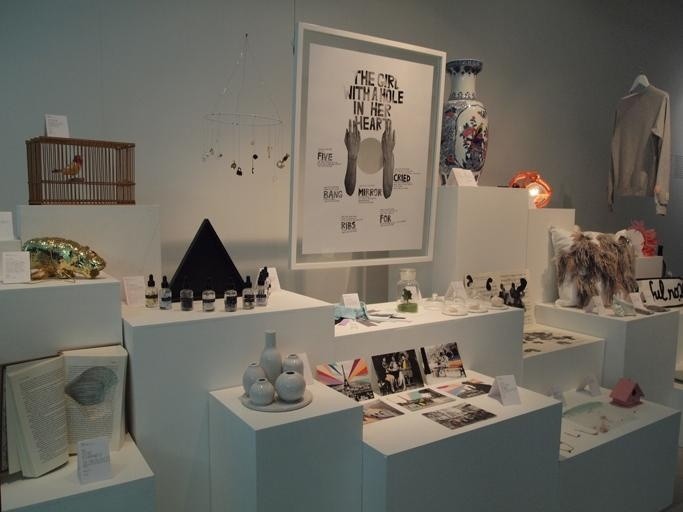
624,67,656,95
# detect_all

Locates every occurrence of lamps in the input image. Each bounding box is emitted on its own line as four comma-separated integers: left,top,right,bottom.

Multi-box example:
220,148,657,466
508,171,555,209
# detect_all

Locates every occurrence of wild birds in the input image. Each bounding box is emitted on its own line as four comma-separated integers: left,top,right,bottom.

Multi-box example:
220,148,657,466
52,154,84,182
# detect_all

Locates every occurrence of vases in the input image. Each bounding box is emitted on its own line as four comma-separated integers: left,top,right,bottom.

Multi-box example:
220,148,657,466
439,58,488,183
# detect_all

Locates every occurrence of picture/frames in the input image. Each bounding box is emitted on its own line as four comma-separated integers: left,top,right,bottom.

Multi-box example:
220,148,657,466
289,22,447,272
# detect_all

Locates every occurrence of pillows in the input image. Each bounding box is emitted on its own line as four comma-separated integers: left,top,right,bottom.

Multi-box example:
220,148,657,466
549,227,639,308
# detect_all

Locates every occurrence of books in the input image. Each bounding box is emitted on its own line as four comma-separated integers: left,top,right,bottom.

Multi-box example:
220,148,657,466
0,346,127,477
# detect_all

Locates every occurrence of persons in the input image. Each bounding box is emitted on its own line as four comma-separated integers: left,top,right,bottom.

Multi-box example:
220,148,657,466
343,116,406,198
390,358,402,388
381,357,396,392
399,354,412,385
